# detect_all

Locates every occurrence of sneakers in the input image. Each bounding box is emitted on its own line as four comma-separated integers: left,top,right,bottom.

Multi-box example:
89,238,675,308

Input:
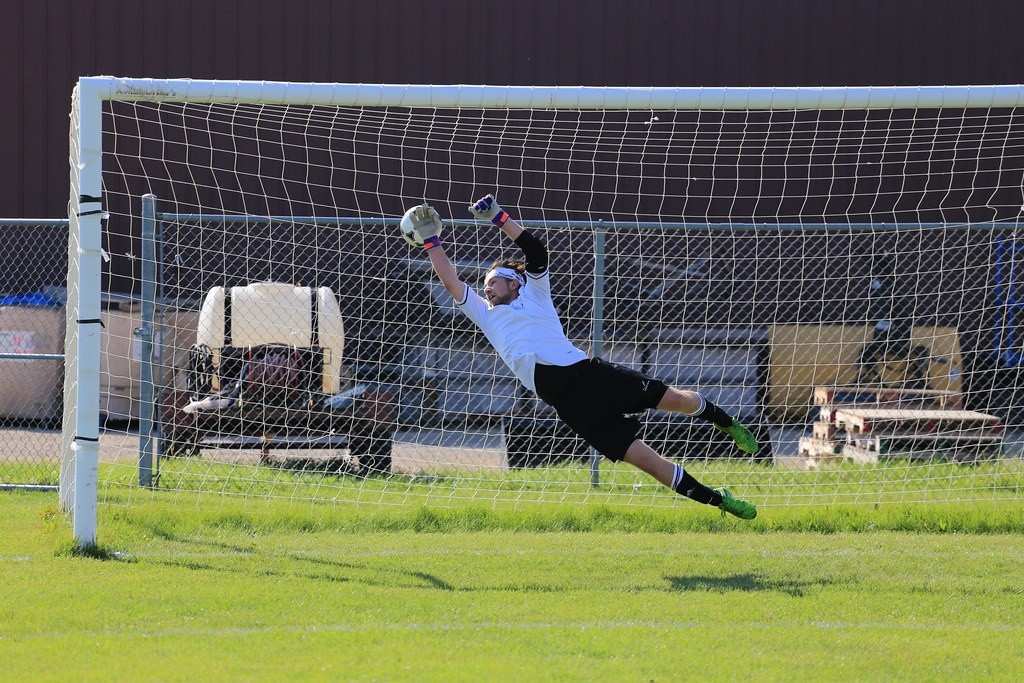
713,416,759,453
713,486,757,520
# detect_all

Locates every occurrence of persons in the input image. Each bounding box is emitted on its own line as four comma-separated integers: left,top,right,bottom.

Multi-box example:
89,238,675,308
409,194,759,520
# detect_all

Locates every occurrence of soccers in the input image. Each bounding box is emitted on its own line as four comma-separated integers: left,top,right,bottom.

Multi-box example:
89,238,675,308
401,206,443,248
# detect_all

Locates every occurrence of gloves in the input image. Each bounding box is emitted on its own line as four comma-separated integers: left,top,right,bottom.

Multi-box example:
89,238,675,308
468,193,510,228
409,203,442,251
